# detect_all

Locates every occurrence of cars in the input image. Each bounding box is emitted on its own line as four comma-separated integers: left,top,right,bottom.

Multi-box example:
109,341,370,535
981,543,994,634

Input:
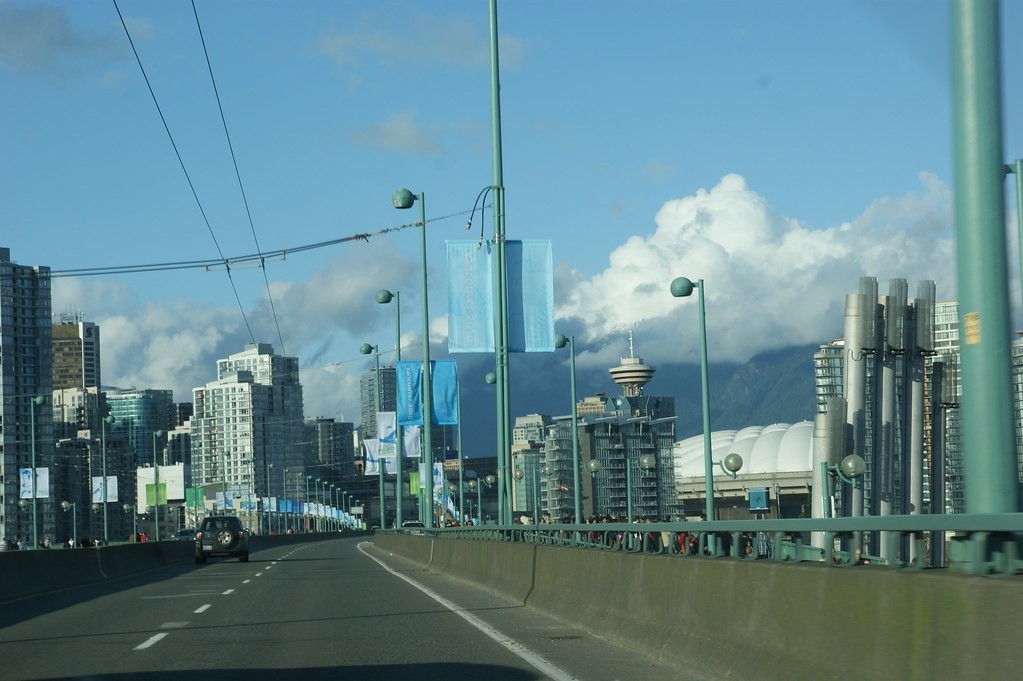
171,528,198,540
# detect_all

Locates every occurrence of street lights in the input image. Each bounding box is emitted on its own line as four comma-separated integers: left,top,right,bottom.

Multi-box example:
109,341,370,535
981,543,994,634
122,504,136,544
532,464,554,537
102,414,116,545
359,343,385,532
667,277,719,556
820,454,868,566
553,334,584,543
296,472,302,531
476,475,495,526
283,468,290,534
315,478,360,531
221,450,231,516
31,395,47,550
152,430,165,541
307,475,312,532
267,464,273,535
465,480,477,521
625,454,656,549
247,457,254,529
60,502,77,548
393,188,433,529
377,289,403,530
450,485,457,519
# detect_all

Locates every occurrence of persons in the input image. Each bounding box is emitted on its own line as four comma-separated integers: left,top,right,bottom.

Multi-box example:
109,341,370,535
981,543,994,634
63,535,108,548
129,530,147,543
39,536,52,549
0,537,27,551
466,513,871,566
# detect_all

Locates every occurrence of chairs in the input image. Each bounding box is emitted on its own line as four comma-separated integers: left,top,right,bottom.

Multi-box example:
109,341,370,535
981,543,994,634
210,522,217,529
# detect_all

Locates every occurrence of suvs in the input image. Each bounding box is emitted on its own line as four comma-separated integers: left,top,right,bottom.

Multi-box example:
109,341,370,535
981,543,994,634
402,519,428,536
195,515,250,565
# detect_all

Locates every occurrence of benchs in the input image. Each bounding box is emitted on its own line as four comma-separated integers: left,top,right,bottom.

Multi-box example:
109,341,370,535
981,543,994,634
0,544,18,552
